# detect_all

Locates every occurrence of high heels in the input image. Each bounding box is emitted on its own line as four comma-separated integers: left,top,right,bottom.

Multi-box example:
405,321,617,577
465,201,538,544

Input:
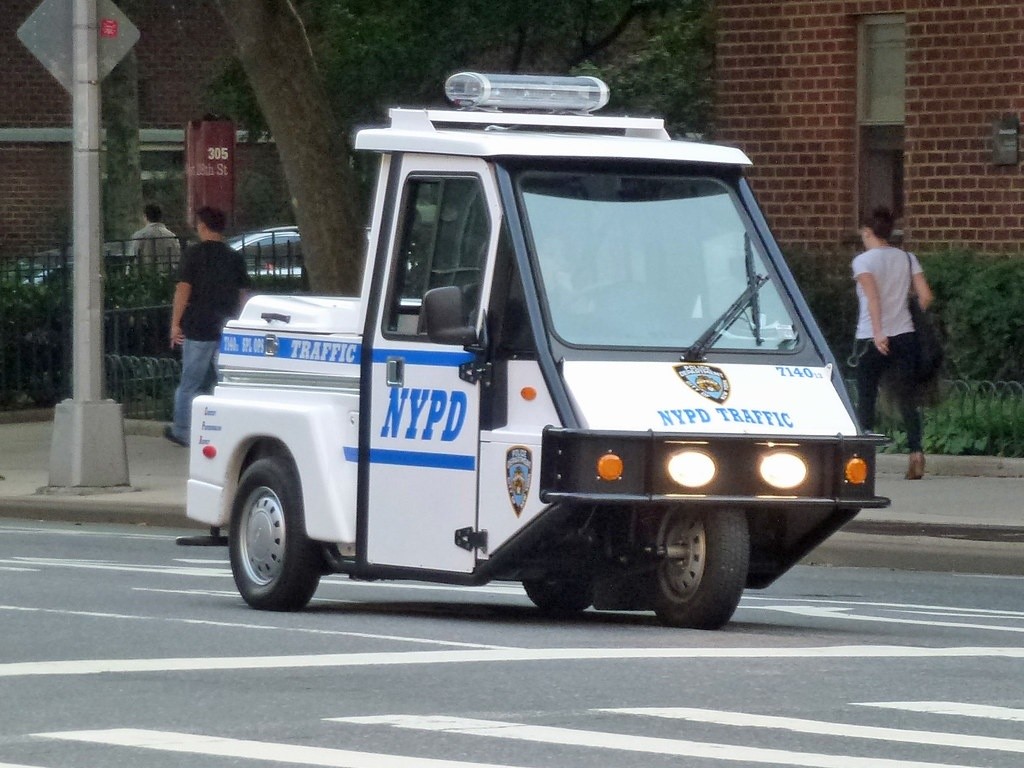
904,451,926,480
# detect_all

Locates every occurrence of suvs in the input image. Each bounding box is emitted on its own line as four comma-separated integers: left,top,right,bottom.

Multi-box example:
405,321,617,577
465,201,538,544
224,226,417,285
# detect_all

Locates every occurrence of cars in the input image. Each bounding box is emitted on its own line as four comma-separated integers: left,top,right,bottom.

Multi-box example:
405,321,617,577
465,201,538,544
5,244,131,291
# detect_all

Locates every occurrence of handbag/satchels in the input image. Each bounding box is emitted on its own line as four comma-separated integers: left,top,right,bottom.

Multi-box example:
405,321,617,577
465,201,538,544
905,251,943,385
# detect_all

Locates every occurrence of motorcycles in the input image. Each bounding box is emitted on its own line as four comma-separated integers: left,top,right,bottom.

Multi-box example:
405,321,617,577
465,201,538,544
172,81,893,627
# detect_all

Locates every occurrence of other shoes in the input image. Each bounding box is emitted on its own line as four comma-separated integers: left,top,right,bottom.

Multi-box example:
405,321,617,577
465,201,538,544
163,426,189,447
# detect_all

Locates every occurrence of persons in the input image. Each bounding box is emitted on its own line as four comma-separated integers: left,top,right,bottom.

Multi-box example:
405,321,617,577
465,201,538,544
125,204,182,284
852,206,931,479
163,209,250,447
